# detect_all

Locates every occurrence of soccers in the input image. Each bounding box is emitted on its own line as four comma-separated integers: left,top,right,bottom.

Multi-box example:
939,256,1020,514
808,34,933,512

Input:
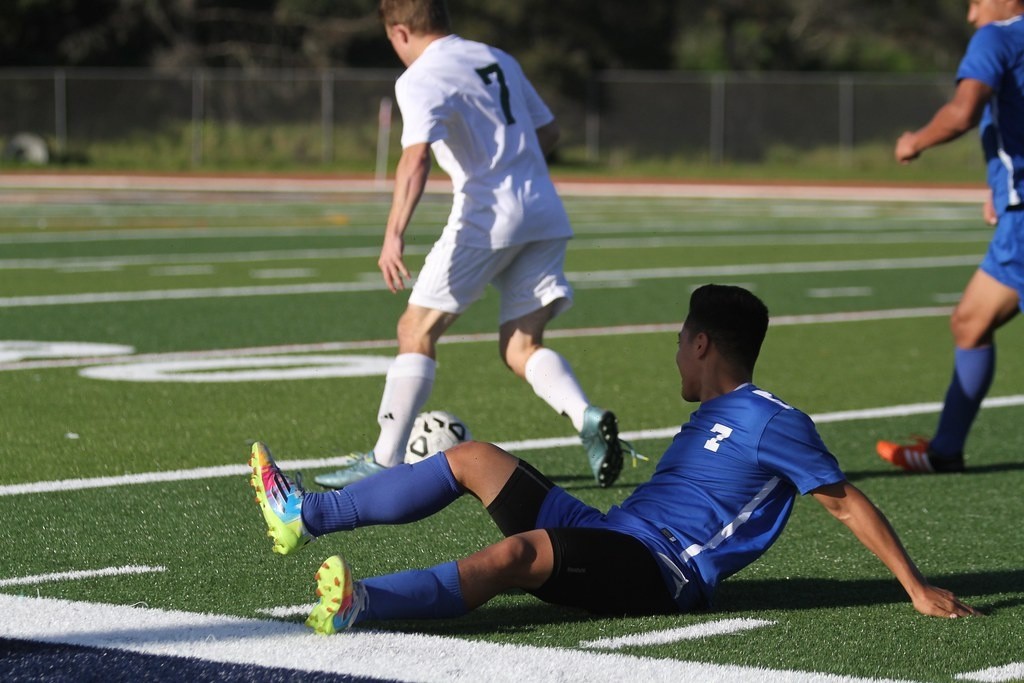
402,409,469,464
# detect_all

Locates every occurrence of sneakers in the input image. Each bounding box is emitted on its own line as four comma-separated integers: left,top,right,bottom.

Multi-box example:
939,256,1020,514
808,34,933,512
577,405,649,488
304,554,366,634
248,441,316,556
313,451,405,488
877,434,933,474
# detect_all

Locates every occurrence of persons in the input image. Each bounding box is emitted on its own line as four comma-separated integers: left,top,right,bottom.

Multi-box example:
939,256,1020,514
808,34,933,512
251,283,984,635
314,1,649,489
876,0,1024,472
